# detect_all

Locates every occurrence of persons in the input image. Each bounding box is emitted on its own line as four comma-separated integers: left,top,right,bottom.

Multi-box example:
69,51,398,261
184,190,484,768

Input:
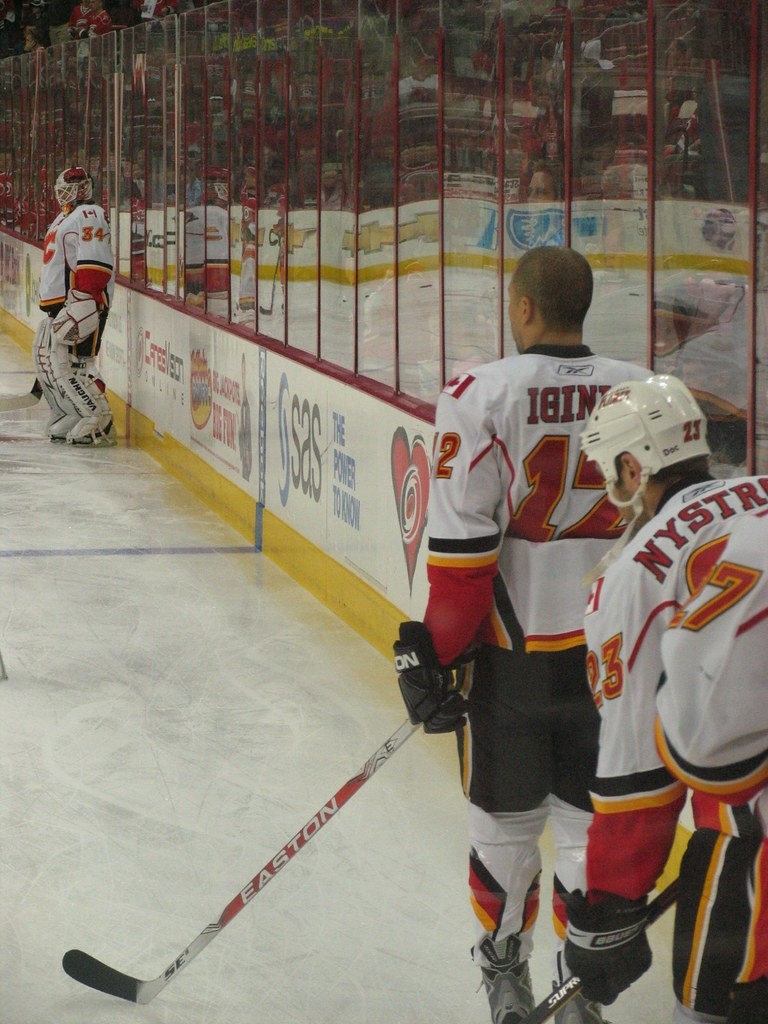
0,0,709,240
35,168,120,444
395,249,653,1024
559,374,768,1023
124,166,754,420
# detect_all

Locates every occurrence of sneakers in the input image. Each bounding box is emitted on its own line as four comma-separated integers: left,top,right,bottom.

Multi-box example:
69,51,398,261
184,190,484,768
470,933,535,1024
552,951,612,1024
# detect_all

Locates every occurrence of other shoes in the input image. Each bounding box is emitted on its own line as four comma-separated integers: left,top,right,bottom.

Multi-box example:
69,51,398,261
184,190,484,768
49,411,78,442
66,416,120,446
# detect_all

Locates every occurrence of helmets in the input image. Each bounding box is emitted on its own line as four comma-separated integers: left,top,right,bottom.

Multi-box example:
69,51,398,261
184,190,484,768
579,375,711,484
57,167,94,200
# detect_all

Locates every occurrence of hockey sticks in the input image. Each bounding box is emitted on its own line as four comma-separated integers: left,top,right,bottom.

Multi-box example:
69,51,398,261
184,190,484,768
513,872,691,1024
64,712,423,1009
0,378,45,413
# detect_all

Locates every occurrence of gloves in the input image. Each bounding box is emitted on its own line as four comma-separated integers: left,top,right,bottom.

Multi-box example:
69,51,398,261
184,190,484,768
393,620,471,735
564,889,653,1006
